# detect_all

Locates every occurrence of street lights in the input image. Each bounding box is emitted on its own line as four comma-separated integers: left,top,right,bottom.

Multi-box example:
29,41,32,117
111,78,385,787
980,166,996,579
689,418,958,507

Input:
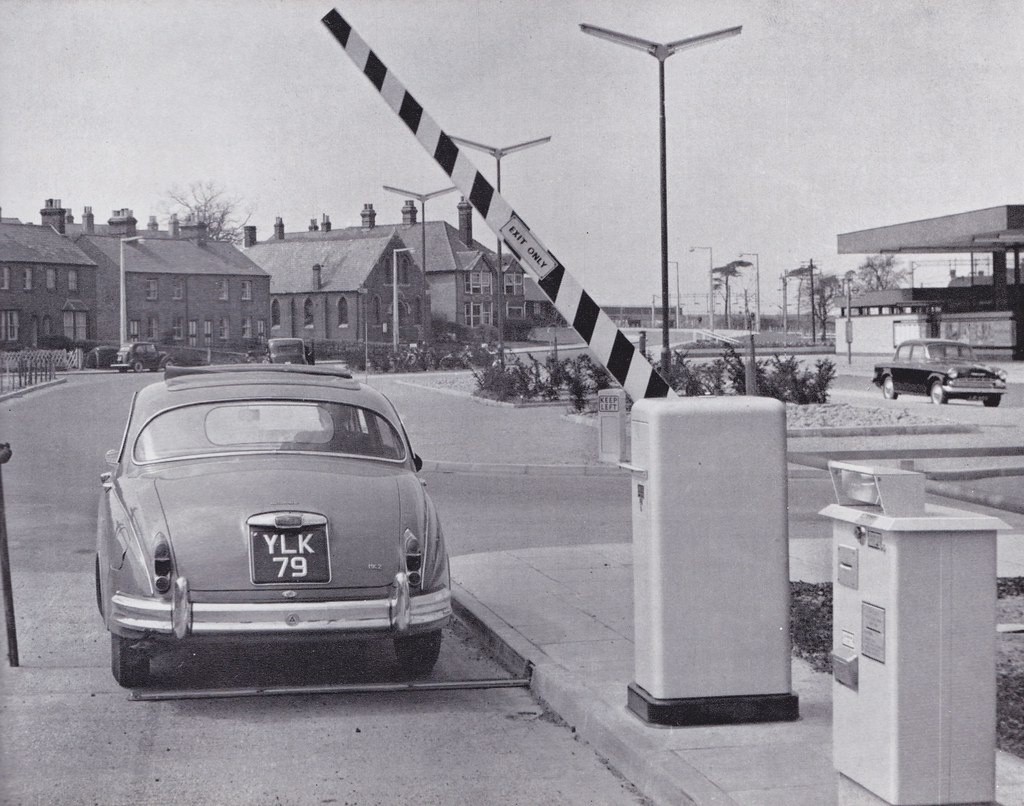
736,253,760,334
580,23,742,383
446,135,552,370
689,247,714,332
846,271,856,364
119,236,149,346
382,184,456,344
392,247,419,353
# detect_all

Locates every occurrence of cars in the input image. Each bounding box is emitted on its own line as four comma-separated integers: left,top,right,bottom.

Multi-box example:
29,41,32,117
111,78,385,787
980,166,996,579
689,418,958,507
114,343,175,372
260,339,308,367
95,364,453,688
871,339,1008,407
